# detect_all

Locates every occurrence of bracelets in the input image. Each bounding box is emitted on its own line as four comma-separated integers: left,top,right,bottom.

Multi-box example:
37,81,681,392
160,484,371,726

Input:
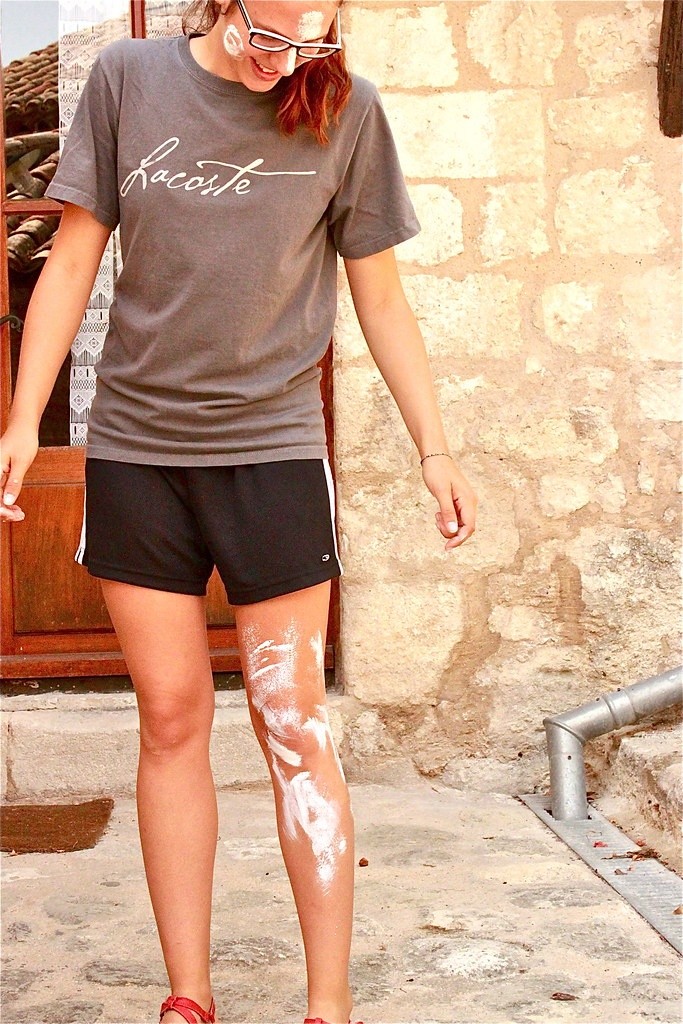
421,454,452,465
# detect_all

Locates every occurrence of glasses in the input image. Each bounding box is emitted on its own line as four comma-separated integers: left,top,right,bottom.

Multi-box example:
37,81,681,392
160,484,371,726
237,0,342,59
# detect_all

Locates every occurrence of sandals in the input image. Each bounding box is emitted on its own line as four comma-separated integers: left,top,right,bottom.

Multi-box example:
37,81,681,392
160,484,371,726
304,1018,363,1024
159,995,216,1024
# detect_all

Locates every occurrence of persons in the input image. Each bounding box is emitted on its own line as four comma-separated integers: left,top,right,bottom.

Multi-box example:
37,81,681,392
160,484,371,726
0,0,478,1024
